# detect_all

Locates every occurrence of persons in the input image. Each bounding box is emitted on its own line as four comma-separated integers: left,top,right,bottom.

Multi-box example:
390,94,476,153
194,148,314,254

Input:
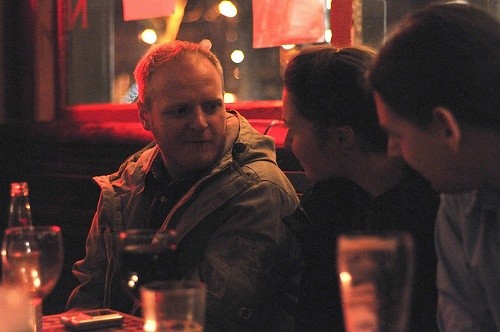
65,3,500,332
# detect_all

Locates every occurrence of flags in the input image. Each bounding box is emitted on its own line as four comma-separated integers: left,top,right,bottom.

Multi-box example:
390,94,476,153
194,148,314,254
122,0,176,22
251,0,325,49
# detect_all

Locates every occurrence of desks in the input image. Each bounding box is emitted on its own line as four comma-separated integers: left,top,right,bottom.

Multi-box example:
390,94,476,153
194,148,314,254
41,308,144,332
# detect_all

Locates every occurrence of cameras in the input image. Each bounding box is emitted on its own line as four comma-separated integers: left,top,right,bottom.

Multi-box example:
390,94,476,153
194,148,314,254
62,308,123,330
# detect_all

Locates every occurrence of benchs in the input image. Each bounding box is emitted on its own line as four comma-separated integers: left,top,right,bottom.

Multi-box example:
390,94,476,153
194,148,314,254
29,157,311,311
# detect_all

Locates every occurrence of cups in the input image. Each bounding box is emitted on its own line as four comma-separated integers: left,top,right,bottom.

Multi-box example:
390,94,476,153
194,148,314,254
117,227,177,309
2,226,65,332
338,230,413,332
140,279,208,332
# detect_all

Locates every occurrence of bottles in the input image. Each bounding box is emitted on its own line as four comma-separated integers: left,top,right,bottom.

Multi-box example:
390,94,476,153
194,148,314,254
3,182,42,332
